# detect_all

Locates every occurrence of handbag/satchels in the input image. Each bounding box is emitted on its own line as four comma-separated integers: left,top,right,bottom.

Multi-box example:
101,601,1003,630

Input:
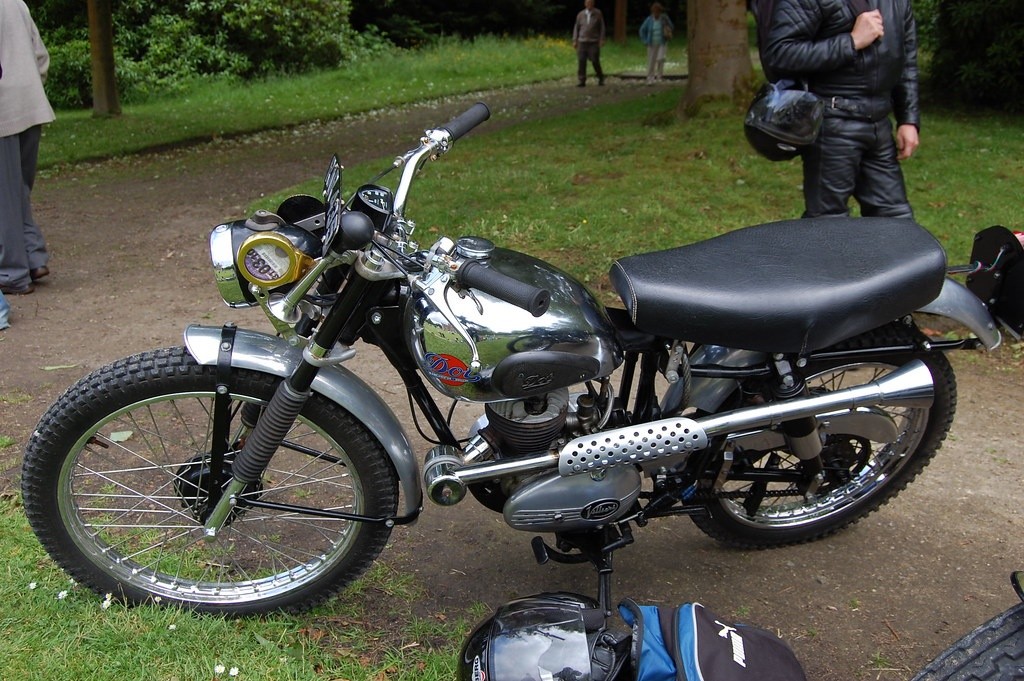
663,25,673,41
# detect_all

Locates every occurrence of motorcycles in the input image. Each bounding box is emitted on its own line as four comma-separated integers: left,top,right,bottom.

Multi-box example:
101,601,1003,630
15,101,1024,616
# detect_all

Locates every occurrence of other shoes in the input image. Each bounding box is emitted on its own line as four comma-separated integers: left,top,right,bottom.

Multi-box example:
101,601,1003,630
657,79,662,84
578,83,586,88
647,80,652,85
0,282,34,294
598,79,604,86
30,266,49,279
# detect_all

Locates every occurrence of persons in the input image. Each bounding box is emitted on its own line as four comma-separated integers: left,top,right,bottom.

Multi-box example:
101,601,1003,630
572,0,606,86
758,0,922,219
0,0,56,331
638,2,675,86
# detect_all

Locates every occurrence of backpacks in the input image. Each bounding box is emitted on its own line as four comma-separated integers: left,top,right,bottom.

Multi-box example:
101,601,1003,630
617,596,807,681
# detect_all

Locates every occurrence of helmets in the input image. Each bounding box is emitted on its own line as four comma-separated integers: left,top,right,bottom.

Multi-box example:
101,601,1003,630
744,82,823,161
456,590,607,681
